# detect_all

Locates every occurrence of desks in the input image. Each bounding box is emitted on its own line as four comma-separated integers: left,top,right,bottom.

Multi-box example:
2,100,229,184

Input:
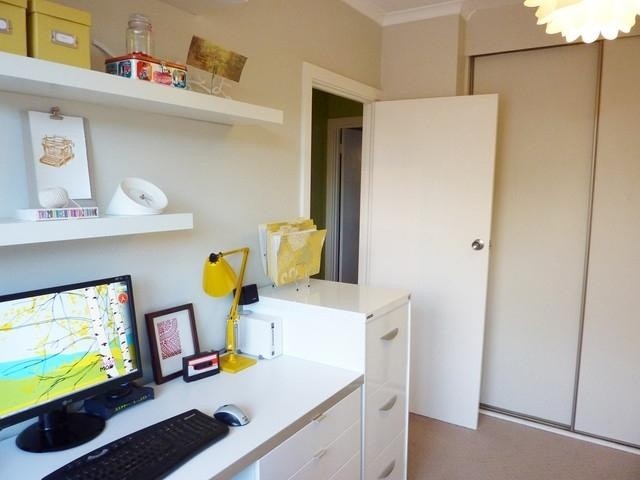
1,346,365,480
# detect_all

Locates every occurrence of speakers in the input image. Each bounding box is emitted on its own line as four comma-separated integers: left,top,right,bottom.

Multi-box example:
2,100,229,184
233,283,259,305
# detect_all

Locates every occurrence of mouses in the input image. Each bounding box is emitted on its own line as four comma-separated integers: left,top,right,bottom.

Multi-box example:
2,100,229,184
213,404,249,426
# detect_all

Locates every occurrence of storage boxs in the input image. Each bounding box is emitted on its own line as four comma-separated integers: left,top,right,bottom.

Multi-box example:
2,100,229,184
0,0,92,70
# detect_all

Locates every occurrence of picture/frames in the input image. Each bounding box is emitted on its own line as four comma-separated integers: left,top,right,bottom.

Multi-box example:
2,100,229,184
143,302,201,386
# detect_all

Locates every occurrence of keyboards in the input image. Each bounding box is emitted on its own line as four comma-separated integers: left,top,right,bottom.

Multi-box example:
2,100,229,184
40,408,229,480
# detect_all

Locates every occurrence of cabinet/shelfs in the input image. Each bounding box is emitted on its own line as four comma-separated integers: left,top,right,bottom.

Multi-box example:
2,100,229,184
241,276,415,480
0,47,286,248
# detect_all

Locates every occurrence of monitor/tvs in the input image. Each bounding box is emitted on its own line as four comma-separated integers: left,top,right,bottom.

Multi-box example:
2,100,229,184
0,275,143,453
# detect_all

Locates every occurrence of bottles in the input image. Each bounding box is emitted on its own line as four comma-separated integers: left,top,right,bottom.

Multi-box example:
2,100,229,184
124,13,154,55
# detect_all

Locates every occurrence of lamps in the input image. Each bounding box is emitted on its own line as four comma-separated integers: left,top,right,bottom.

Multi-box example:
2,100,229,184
201,248,259,374
521,0,640,46
